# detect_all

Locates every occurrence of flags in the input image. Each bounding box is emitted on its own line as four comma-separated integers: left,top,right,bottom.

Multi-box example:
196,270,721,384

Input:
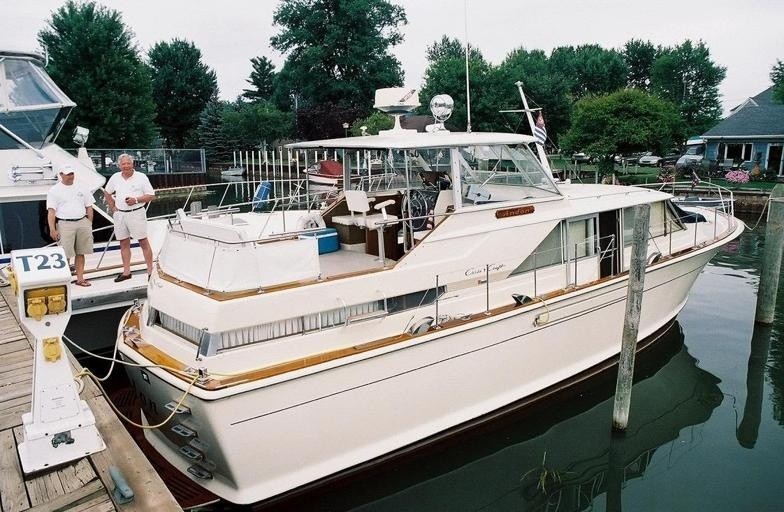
533,112,548,148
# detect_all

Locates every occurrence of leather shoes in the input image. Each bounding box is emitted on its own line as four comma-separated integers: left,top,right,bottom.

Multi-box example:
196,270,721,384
115,274,131,281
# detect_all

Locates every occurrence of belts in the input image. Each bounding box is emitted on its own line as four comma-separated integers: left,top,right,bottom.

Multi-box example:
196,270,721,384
59,217,85,221
120,207,142,212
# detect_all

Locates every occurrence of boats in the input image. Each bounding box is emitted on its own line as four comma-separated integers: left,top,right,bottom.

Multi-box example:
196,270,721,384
114,85,746,504
219,165,247,177
274,314,736,510
0,44,106,255
3,175,357,370
302,159,359,186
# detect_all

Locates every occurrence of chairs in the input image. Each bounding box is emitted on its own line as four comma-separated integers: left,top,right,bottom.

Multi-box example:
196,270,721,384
342,189,398,267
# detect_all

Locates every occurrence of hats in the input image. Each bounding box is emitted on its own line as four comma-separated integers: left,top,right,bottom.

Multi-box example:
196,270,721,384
59,165,75,174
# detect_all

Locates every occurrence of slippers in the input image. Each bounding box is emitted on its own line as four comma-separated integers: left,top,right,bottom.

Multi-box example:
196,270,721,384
76,280,91,286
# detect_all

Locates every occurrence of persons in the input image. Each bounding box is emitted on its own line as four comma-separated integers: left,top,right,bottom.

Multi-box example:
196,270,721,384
47,167,96,287
104,155,155,282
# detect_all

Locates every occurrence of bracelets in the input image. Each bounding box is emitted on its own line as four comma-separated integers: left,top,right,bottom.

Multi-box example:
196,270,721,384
134,197,139,204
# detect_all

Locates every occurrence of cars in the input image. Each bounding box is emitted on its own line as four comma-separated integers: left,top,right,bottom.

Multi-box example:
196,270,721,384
571,151,702,167
